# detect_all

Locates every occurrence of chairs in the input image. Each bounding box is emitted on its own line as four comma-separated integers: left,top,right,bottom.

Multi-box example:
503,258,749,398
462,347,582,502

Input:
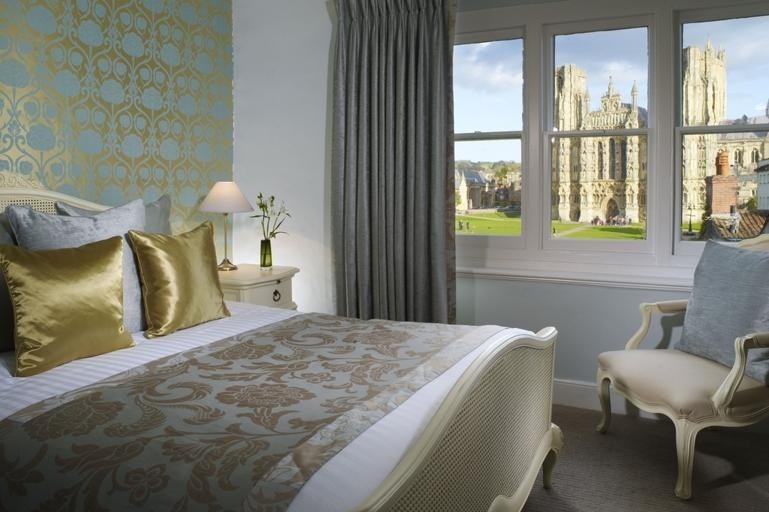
597,233,768,499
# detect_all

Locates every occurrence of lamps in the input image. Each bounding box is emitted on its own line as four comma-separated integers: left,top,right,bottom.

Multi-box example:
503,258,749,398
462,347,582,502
197,181,254,271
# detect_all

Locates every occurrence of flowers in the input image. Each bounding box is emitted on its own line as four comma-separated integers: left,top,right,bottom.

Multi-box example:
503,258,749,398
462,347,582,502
249,192,291,264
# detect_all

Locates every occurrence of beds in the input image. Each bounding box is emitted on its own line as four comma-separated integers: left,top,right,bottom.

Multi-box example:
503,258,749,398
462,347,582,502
0,168,565,512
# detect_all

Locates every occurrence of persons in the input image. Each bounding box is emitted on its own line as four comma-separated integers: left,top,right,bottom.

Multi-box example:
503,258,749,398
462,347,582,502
591,215,633,226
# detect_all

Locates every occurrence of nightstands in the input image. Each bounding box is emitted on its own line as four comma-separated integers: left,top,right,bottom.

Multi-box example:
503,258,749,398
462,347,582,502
218,262,300,312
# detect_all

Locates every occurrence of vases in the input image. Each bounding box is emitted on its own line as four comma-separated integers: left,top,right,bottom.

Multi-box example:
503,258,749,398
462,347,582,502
259,239,272,267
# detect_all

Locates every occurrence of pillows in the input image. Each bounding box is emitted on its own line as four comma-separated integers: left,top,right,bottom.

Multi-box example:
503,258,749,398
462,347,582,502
677,240,768,384
